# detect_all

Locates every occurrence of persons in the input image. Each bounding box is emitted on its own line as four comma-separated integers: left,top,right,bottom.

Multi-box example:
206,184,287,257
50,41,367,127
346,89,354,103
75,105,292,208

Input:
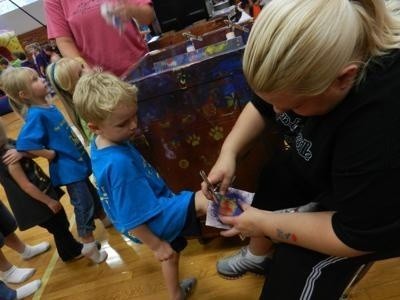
200,0,400,299
0,35,114,299
72,64,316,300
43,1,157,81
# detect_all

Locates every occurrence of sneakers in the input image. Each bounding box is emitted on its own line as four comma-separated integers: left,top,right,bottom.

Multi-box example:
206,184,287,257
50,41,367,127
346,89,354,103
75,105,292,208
216,245,273,280
